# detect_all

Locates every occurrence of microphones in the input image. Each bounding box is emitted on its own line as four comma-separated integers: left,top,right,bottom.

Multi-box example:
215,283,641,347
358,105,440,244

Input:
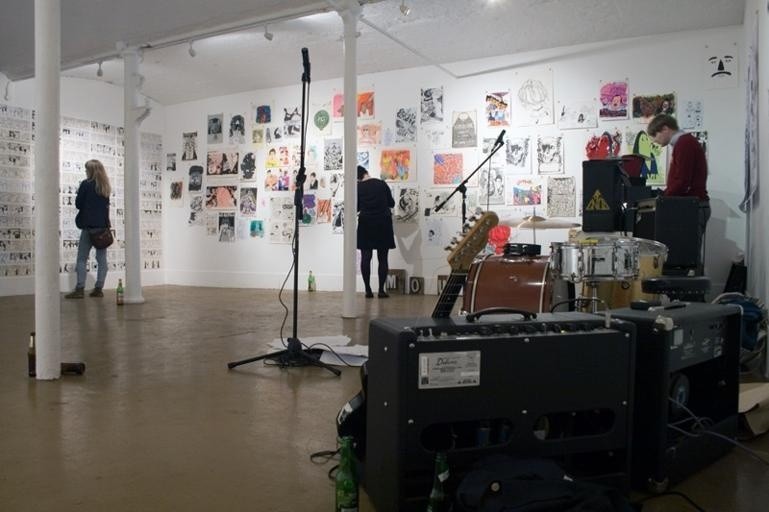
495,128,511,149
301,46,314,77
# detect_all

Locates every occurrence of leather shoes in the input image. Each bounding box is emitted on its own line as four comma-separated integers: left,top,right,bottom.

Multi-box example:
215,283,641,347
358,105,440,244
378,292,388,297
366,292,373,298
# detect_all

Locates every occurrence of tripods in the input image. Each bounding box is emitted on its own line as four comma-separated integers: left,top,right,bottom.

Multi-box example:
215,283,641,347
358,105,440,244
227,90,343,377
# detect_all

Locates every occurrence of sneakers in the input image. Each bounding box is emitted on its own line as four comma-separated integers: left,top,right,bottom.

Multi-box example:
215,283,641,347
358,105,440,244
90,289,102,296
66,289,83,298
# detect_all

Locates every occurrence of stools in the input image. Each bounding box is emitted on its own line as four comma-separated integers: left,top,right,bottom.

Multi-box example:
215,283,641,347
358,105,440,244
641,277,711,306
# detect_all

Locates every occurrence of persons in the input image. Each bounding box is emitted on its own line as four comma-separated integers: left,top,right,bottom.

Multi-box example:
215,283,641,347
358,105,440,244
266,149,278,167
357,166,395,298
281,171,291,189
495,176,502,193
649,115,711,270
310,173,317,190
65,159,110,298
265,170,276,187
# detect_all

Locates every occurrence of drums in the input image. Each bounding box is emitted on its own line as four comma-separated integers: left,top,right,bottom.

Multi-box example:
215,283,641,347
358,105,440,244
578,237,669,314
458,242,570,313
551,235,637,282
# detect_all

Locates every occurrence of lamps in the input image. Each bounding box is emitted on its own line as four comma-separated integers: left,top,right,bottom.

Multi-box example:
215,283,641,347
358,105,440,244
399,0,410,16
97,60,103,76
188,25,273,57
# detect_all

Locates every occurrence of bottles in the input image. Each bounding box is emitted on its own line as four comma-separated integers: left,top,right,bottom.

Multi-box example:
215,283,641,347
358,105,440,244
61,362,85,376
28,333,35,377
307,270,314,292
334,435,360,512
116,278,124,305
427,453,455,512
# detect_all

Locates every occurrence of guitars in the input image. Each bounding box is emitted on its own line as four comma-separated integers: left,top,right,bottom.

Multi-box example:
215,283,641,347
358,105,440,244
337,205,498,442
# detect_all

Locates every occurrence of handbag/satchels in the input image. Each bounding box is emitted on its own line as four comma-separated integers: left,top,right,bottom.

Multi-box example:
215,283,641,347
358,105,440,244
90,227,113,249
